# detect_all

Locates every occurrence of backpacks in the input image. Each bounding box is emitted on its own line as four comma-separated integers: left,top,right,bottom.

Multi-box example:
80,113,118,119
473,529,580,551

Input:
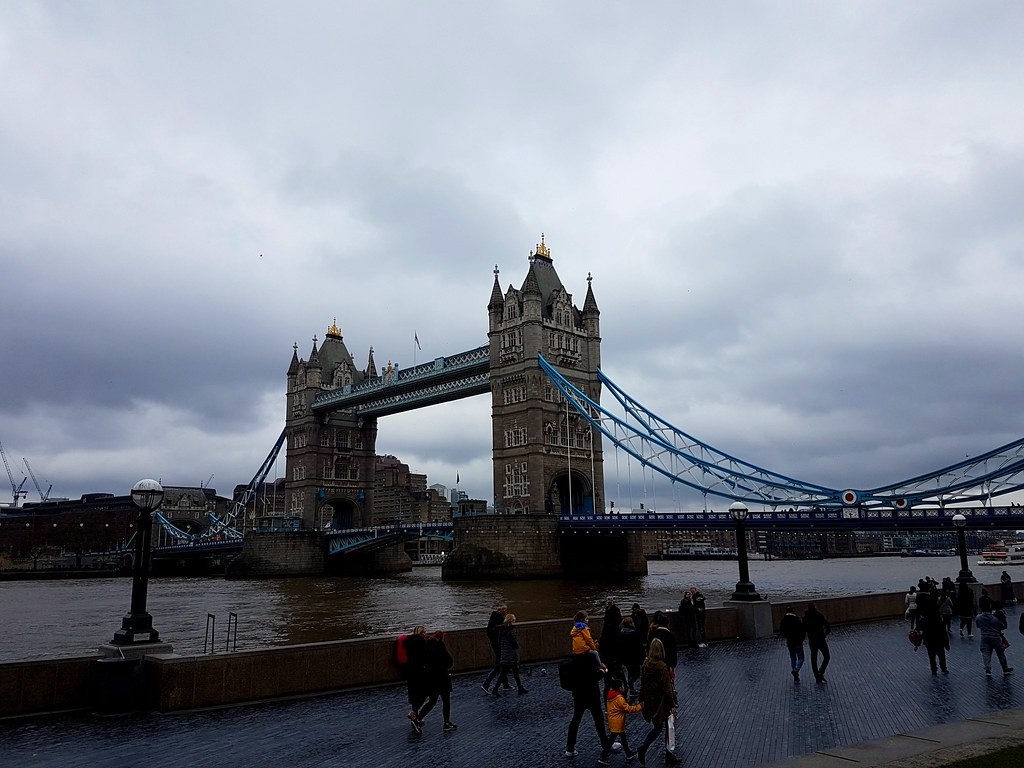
558,659,581,691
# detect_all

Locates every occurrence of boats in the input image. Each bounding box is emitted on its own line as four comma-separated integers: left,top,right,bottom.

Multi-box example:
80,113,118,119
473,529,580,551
977,534,1024,566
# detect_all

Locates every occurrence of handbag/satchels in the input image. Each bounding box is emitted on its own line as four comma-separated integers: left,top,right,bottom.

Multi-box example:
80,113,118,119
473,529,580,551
667,713,675,753
908,630,922,647
1000,634,1010,651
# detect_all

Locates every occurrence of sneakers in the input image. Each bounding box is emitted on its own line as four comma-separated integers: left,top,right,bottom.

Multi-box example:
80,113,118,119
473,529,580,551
411,719,422,734
503,685,515,691
517,687,528,694
443,723,458,731
481,685,493,695
492,688,502,698
408,711,417,722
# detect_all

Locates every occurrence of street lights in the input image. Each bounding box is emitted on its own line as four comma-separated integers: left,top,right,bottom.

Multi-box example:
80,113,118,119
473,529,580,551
951,513,983,587
98,477,174,658
723,502,771,607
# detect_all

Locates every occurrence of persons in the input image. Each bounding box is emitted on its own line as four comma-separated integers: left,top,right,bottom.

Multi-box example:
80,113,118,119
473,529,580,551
916,609,950,676
401,627,458,734
904,575,993,638
480,604,528,697
975,601,1014,676
1000,570,1011,604
678,587,708,647
565,599,681,768
802,601,831,683
779,607,806,682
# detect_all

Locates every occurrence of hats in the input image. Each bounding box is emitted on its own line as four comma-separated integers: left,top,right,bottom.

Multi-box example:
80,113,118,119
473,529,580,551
575,610,588,621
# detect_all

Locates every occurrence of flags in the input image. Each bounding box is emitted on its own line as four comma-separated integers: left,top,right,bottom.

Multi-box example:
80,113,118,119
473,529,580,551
415,334,421,350
457,474,459,484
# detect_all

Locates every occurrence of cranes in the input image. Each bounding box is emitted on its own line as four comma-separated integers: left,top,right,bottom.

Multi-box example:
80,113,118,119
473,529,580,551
21,457,53,503
205,473,215,487
0,441,29,507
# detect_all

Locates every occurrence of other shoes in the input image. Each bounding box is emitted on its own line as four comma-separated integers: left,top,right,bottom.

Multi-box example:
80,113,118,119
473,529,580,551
959,629,964,637
985,670,991,675
967,635,974,638
794,679,800,685
790,670,801,681
563,744,681,766
820,676,827,684
1002,668,1014,674
815,673,823,684
